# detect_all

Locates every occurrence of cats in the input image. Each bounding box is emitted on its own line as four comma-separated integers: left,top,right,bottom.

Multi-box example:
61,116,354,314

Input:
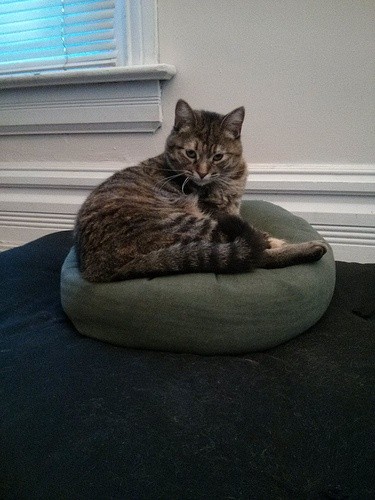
73,99,328,286
